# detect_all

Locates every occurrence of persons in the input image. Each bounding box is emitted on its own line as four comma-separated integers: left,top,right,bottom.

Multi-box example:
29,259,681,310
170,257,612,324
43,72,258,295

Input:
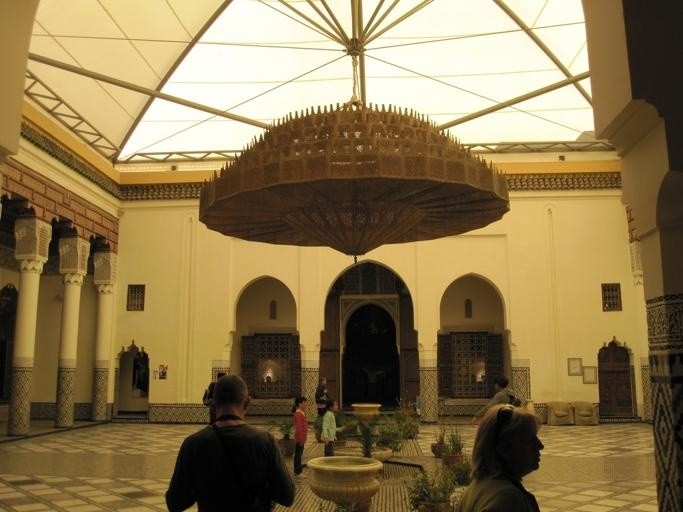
315,377,329,416
165,374,295,512
471,376,521,425
321,400,339,456
292,397,307,477
457,403,544,511
203,373,226,425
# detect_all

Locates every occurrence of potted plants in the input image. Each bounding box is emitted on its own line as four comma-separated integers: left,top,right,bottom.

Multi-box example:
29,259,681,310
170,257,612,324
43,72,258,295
314,416,324,442
265,416,296,457
334,411,346,449
408,418,464,512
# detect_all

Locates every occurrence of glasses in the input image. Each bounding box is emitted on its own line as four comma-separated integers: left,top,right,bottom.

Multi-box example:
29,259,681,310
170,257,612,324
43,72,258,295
497,405,512,436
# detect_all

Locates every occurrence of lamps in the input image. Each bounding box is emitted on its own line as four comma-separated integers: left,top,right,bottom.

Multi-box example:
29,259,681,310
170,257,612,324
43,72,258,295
199,55,511,266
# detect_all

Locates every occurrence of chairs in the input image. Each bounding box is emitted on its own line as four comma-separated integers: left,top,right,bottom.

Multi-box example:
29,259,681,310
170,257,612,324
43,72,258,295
571,401,599,426
547,401,575,426
